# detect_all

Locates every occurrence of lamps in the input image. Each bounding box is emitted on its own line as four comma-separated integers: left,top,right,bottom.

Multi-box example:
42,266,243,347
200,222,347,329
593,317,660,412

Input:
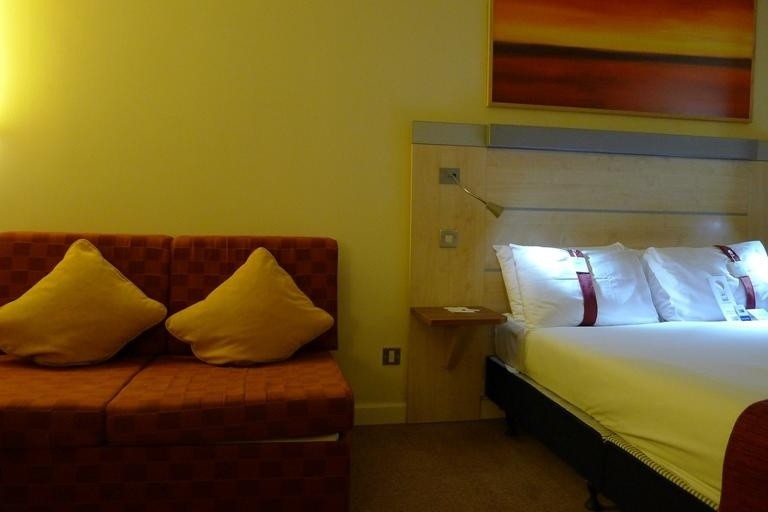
439,168,505,218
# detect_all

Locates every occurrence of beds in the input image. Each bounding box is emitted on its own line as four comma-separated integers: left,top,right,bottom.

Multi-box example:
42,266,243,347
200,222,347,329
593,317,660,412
485,313,768,512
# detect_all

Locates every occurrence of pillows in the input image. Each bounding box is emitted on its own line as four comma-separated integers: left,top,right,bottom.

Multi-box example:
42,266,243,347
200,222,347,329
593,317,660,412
492,239,768,334
2,239,166,367
166,246,334,367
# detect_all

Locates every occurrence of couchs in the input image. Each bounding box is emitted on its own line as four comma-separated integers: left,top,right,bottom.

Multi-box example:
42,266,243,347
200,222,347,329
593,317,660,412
0,231,353,511
